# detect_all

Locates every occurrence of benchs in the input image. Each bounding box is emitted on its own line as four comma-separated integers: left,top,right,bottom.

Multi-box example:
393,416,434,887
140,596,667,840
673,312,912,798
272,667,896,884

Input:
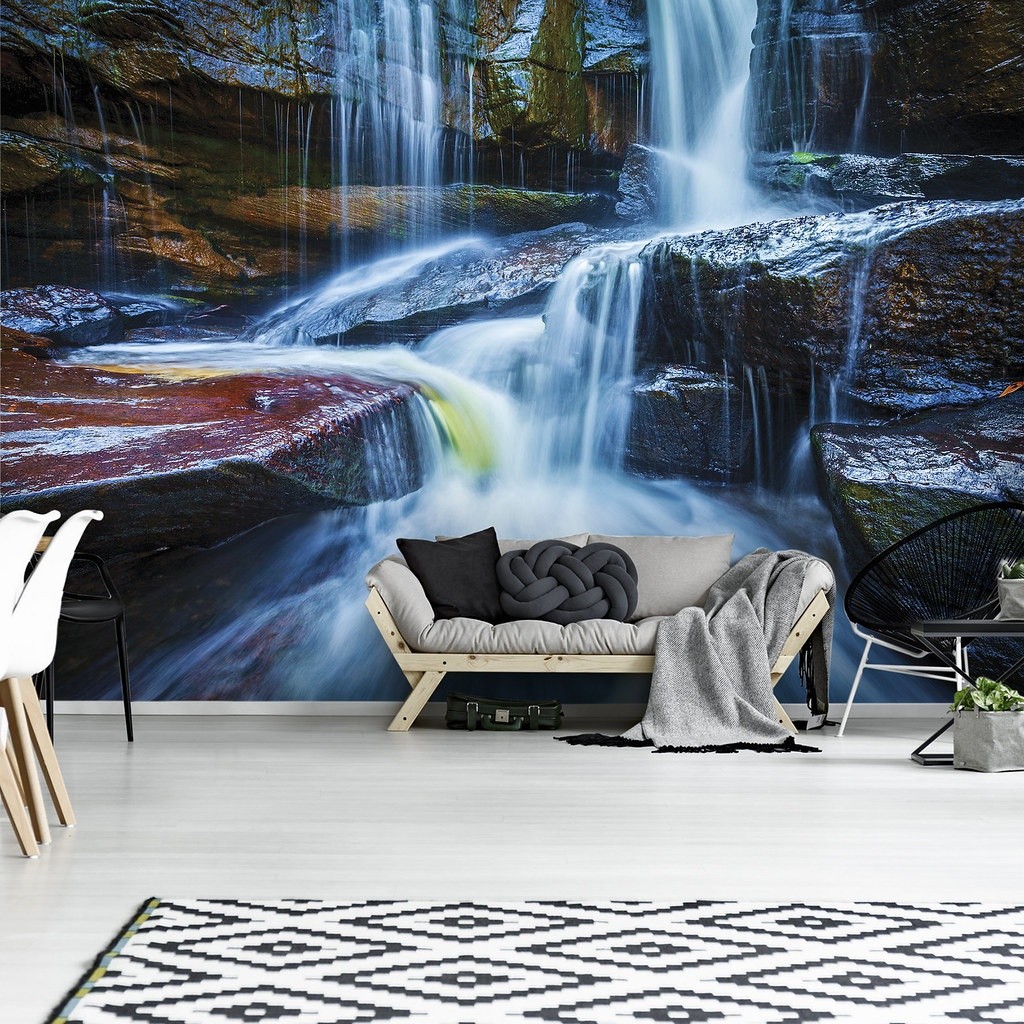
363,549,834,734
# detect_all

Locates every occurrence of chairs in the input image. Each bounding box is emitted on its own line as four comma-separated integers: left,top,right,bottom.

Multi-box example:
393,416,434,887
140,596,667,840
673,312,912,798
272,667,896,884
30,537,134,746
0,509,62,859
0,510,104,849
833,501,1024,738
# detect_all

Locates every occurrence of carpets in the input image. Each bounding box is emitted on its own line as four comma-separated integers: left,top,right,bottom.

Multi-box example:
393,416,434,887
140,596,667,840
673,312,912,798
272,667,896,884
41,895,1024,1024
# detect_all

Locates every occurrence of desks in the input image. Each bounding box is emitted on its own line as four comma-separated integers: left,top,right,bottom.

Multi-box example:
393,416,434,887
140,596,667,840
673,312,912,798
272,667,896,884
911,619,1024,766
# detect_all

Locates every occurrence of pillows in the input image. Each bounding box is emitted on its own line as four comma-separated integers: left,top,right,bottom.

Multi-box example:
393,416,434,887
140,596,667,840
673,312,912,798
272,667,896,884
434,530,590,555
587,534,735,620
395,526,505,622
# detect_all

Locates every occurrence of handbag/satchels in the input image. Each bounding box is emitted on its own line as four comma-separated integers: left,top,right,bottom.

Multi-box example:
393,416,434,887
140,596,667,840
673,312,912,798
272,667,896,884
444,693,563,731
